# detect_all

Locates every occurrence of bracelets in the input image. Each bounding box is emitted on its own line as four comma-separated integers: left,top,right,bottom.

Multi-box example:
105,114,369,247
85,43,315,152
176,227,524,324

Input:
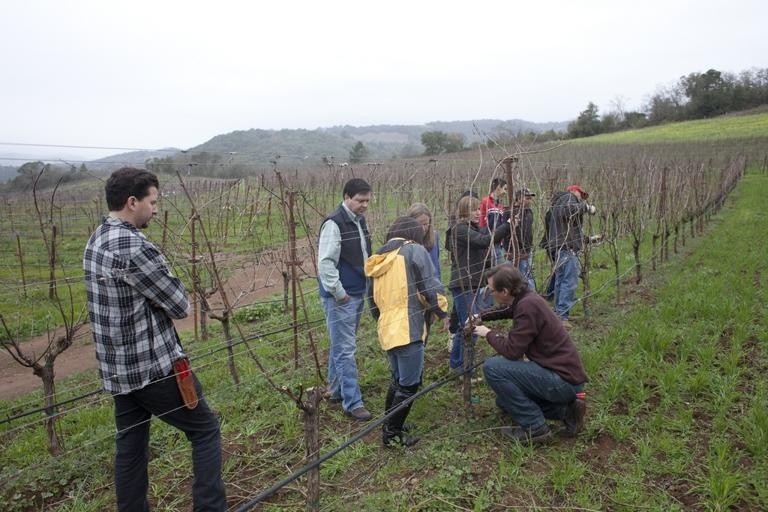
587,205,591,215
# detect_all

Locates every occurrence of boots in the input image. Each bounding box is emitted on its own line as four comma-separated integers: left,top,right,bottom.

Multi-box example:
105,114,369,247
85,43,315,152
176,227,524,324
383,380,420,446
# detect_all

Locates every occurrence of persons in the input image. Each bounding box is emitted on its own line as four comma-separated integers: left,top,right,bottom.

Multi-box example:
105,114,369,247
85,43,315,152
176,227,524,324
446,191,478,354
463,263,589,440
546,185,595,328
407,203,438,284
364,216,447,447
538,204,555,301
512,188,536,290
445,195,519,384
479,177,506,229
83,167,226,512
315,175,371,421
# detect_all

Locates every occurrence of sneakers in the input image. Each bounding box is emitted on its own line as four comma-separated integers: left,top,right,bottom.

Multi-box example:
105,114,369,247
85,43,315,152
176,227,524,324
348,406,371,420
500,424,552,444
559,399,586,437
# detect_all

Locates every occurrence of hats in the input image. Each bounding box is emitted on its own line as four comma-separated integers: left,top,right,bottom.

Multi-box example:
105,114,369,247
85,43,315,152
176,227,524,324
567,186,589,199
516,188,536,196
